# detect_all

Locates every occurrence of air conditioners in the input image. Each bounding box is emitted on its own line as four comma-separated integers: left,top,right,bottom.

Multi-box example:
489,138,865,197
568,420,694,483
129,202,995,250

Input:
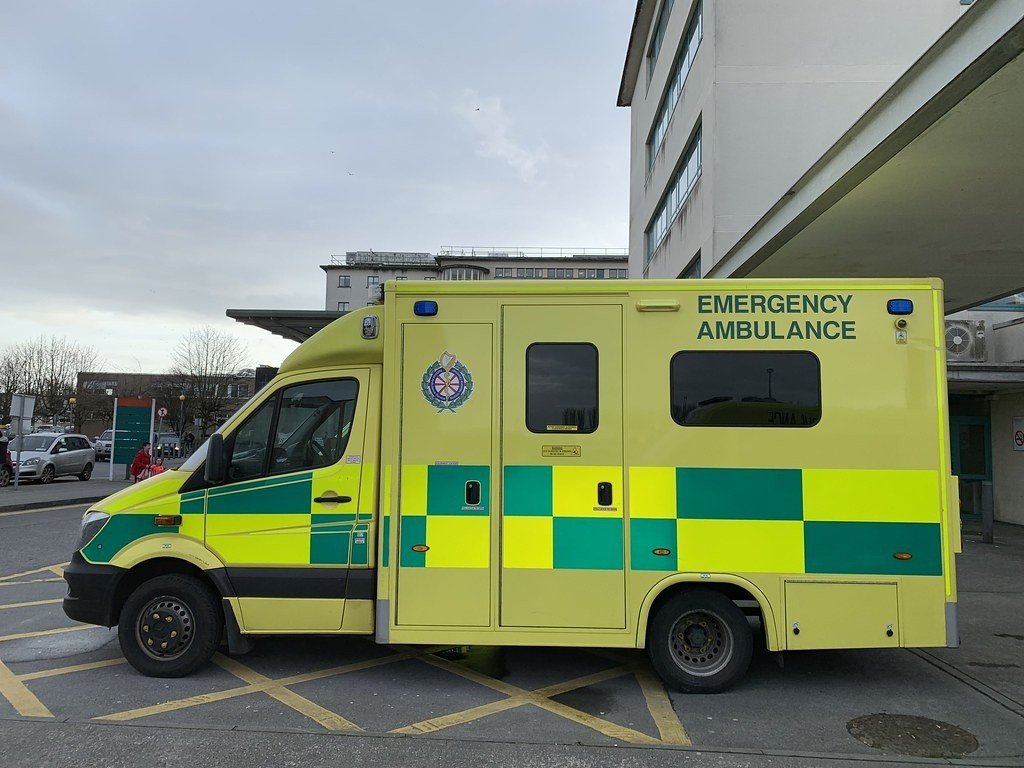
944,318,986,362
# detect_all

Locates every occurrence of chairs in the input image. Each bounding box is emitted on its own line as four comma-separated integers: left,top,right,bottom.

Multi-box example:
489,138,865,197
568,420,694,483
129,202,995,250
44,439,62,453
30,439,42,448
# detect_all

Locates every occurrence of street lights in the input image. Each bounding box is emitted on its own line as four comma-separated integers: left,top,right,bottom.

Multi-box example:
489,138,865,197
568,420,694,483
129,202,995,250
178,395,186,459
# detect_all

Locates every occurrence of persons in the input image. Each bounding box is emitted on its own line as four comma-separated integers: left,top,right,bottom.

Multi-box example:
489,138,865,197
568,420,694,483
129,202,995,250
0,415,12,439
130,442,152,484
150,457,168,477
184,431,194,450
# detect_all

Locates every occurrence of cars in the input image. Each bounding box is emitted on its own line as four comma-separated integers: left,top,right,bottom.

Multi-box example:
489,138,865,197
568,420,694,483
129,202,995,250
157,432,185,458
68,398,75,433
3,431,96,484
0,424,75,487
93,430,130,462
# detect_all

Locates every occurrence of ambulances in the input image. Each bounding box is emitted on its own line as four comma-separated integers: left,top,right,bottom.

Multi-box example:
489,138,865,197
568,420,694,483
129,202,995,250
60,279,964,696
